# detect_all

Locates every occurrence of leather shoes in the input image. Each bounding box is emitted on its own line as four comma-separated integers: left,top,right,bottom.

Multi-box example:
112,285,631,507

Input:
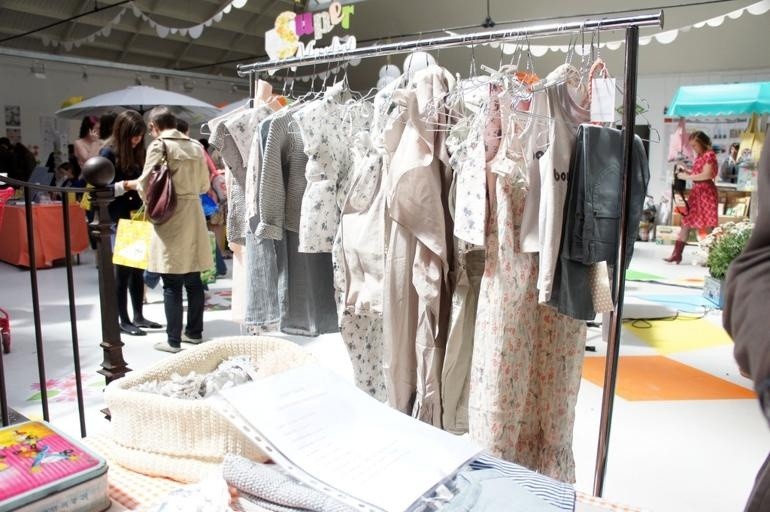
132,319,162,328
120,324,145,335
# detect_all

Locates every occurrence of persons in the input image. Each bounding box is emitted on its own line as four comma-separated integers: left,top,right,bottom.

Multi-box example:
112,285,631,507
97,109,162,336
662,130,719,264
718,118,770,510
719,142,740,183
49,113,231,306
137,107,215,353
1,120,36,190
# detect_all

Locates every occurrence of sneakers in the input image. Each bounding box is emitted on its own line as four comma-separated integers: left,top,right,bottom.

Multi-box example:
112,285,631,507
154,342,180,352
181,334,202,343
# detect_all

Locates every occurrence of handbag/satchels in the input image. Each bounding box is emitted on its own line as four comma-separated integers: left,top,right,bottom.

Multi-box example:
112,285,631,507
200,231,217,284
733,113,766,167
146,163,176,224
674,173,686,191
669,118,693,162
112,205,151,270
210,169,228,200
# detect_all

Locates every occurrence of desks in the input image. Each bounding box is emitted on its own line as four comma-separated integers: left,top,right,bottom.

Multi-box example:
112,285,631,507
1,200,89,271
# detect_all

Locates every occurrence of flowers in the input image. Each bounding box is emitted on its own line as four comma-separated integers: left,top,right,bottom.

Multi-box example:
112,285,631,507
697,212,755,279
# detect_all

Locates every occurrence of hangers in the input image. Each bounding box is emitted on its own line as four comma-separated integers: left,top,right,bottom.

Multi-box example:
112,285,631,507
199,17,660,149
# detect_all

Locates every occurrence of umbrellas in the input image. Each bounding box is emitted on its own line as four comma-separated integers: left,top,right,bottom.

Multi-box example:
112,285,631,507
54,78,226,146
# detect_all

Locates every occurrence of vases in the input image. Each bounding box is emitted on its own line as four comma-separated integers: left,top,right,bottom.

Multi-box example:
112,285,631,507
702,275,725,308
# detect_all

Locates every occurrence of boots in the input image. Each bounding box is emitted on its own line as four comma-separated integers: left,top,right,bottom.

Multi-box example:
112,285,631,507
664,240,685,264
